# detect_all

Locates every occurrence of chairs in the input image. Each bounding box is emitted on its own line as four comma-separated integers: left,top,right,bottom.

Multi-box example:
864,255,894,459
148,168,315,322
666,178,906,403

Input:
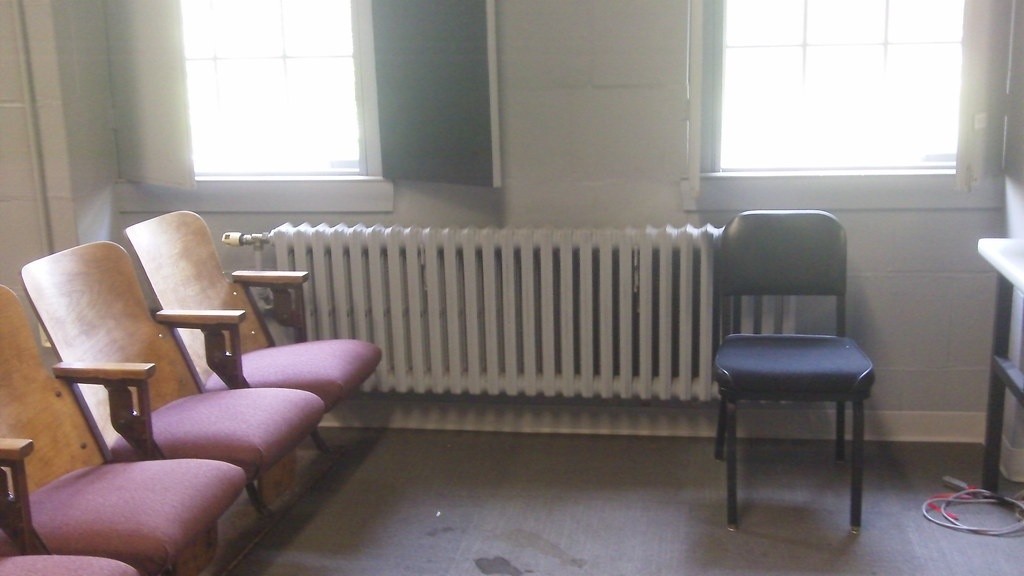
0,286,247,576
123,212,379,458
0,555,141,576
713,210,873,531
20,239,325,515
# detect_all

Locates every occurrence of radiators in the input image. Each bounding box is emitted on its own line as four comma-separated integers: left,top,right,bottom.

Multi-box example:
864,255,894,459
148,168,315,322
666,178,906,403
221,221,794,402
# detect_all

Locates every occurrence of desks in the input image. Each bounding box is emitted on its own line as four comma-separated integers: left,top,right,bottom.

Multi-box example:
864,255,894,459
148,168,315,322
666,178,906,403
978,237,1024,494
